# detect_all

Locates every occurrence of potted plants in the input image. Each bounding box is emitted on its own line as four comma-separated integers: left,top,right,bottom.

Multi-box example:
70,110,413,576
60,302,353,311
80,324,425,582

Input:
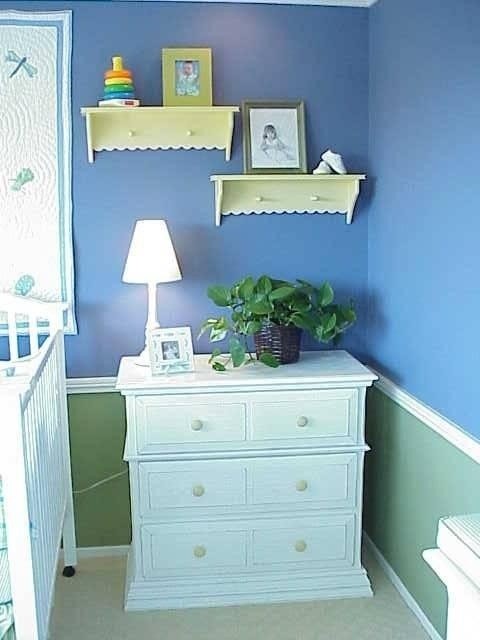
197,274,357,371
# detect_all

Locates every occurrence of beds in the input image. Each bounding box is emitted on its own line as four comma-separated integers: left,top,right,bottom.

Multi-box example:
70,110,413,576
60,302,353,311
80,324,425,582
0,292,77,640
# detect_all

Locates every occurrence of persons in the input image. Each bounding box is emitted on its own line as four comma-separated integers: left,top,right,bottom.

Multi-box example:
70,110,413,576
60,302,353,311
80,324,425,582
176,60,198,95
259,125,294,160
162,342,178,360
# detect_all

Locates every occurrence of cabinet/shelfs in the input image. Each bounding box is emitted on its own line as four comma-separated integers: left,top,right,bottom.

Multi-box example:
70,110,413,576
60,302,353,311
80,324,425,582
115,379,379,612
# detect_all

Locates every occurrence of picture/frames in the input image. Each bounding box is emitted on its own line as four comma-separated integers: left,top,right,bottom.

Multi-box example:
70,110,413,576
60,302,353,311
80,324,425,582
162,48,213,106
148,327,195,377
1,9,78,336
242,100,308,175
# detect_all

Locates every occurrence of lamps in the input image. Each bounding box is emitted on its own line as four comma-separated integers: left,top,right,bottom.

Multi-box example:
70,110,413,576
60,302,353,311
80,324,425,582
121,219,183,367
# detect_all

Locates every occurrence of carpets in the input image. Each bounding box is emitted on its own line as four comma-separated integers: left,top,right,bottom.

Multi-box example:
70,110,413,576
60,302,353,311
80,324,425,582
48,543,434,640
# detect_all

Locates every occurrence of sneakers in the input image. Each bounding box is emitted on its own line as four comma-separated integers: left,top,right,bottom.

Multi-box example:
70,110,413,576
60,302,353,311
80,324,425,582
313,161,331,174
321,150,347,175
